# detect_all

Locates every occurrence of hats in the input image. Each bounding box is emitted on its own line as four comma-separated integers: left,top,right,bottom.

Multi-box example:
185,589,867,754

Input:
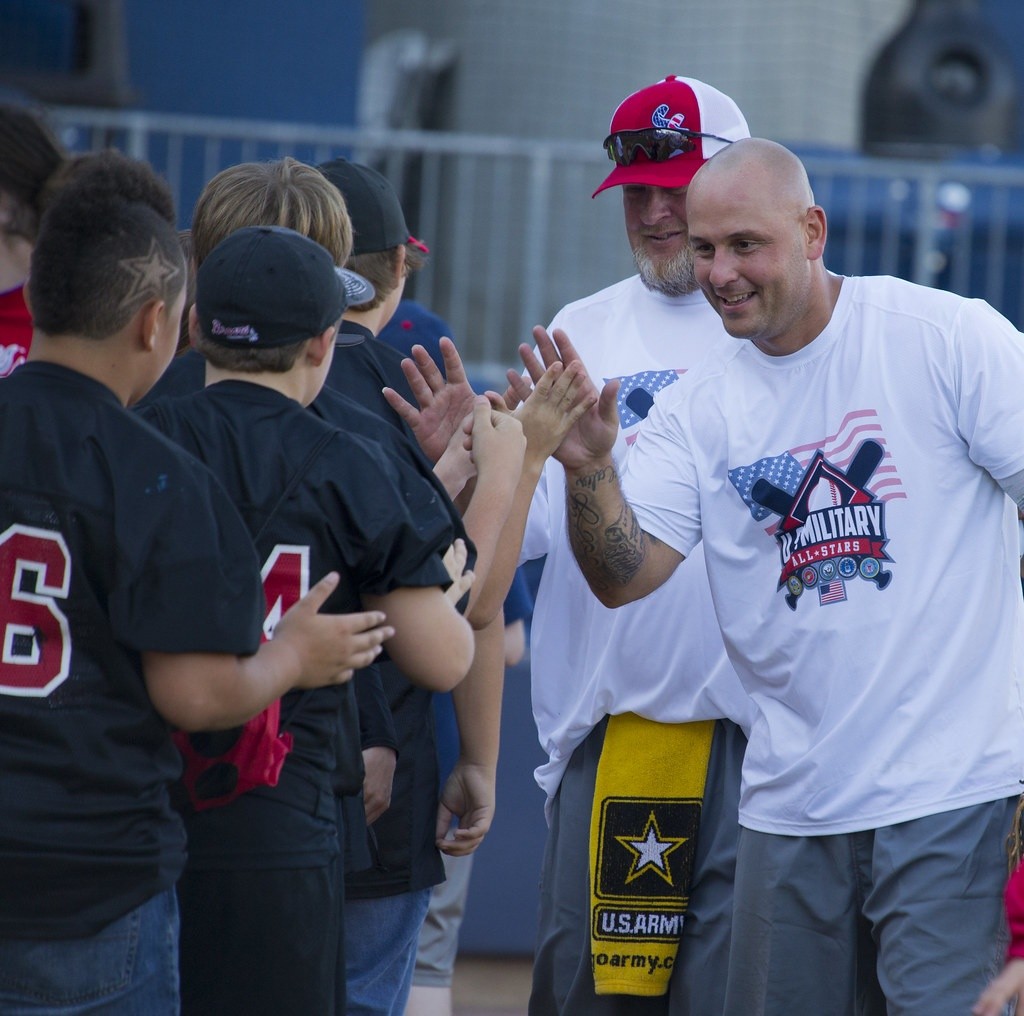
592,75,751,199
316,155,429,256
196,226,375,349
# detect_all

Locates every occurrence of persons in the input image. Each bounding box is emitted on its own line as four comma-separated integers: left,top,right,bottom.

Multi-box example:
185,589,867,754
1,105,596,1016
508,136,1023,1016
381,70,755,1016
973,854,1024,1014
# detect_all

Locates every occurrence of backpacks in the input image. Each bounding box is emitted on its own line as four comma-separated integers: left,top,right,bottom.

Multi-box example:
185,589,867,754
151,393,345,818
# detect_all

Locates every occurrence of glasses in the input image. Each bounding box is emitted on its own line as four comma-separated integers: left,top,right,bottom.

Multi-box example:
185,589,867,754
603,127,733,166
